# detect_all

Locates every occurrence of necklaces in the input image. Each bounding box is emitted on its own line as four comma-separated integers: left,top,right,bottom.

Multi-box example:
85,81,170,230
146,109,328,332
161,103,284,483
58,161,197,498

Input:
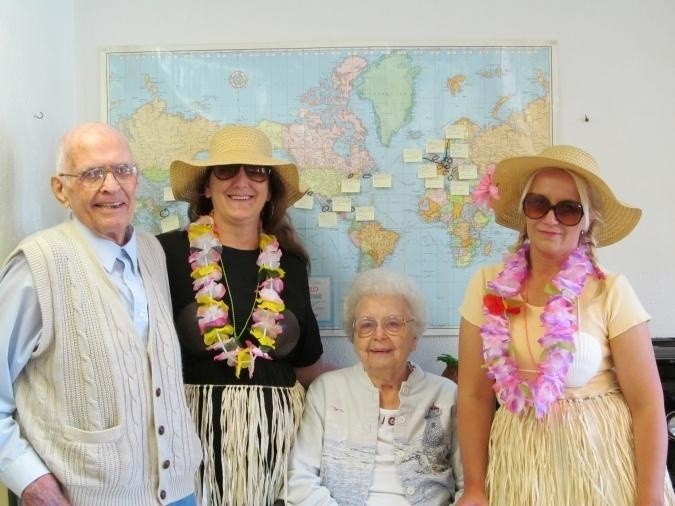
218,255,260,344
522,273,540,368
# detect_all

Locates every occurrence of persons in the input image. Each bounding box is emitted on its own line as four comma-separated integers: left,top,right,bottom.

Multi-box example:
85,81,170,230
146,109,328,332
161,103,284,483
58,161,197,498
454,143,674,505
286,267,467,506
154,123,340,506
0,122,206,506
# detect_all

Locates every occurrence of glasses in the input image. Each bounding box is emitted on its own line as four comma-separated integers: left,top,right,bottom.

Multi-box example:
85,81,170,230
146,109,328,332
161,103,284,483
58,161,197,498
60,163,137,187
213,164,272,182
353,315,414,337
523,193,584,226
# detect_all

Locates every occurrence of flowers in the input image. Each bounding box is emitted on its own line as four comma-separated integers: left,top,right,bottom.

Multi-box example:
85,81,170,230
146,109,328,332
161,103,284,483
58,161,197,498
186,213,289,378
475,240,608,424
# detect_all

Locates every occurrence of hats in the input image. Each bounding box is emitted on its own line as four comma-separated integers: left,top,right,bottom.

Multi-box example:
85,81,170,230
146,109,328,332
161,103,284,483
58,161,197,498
169,125,309,209
491,146,642,248
